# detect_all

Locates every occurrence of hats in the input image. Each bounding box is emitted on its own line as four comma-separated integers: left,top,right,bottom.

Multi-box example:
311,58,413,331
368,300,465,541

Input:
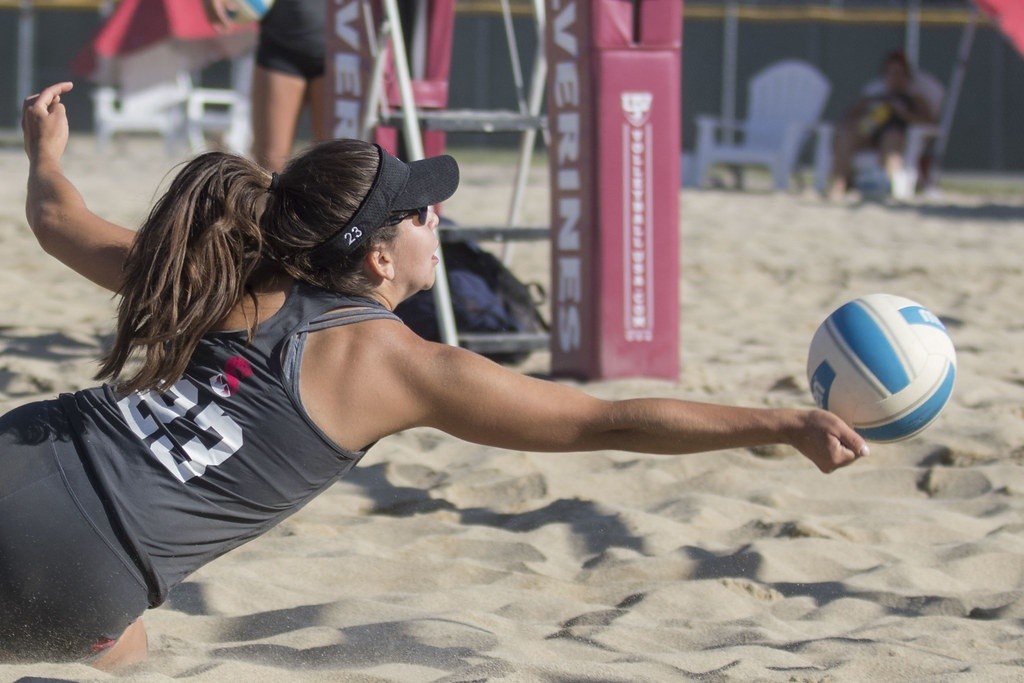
274,144,460,275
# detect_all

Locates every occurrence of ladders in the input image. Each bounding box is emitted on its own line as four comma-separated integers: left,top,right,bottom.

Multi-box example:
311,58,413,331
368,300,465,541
359,1,553,351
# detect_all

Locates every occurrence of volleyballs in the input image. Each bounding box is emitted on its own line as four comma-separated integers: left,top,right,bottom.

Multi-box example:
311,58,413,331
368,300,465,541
806,293,957,442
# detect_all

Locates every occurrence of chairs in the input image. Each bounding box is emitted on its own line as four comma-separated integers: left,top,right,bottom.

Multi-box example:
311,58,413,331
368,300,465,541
88,52,253,171
814,72,945,198
693,60,837,189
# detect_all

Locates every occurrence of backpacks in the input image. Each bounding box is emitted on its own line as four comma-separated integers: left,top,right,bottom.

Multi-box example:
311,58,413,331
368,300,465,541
393,217,551,366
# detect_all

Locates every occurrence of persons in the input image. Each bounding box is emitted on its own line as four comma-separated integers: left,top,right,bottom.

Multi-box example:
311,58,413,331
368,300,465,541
204,0,327,177
828,48,932,202
0,80,867,673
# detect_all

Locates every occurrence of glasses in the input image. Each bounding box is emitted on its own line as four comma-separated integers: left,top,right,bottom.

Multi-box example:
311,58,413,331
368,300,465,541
390,207,428,226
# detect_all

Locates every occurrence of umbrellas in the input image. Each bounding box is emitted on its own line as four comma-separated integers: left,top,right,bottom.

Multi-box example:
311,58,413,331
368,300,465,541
75,0,261,93
